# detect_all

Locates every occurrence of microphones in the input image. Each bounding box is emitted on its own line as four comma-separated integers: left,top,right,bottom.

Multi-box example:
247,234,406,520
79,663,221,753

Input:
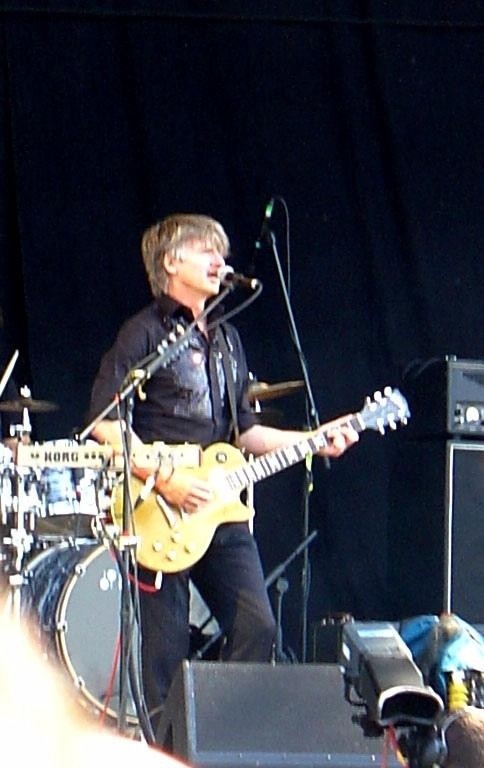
247,198,275,269
217,265,259,290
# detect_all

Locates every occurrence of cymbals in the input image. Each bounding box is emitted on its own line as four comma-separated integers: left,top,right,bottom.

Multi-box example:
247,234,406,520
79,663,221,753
4,401,58,415
243,378,308,404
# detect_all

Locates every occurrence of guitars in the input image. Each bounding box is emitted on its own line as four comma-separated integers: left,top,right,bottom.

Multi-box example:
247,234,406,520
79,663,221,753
108,387,411,574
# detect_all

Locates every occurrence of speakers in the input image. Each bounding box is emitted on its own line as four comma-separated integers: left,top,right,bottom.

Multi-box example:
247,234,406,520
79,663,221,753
155,659,404,768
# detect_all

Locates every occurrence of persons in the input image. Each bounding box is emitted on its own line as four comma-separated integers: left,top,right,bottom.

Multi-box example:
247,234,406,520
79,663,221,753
445,708,484,768
87,214,360,734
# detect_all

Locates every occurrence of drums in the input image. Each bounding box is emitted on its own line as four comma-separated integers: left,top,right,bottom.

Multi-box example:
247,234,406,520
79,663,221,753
21,539,165,725
18,439,115,537
